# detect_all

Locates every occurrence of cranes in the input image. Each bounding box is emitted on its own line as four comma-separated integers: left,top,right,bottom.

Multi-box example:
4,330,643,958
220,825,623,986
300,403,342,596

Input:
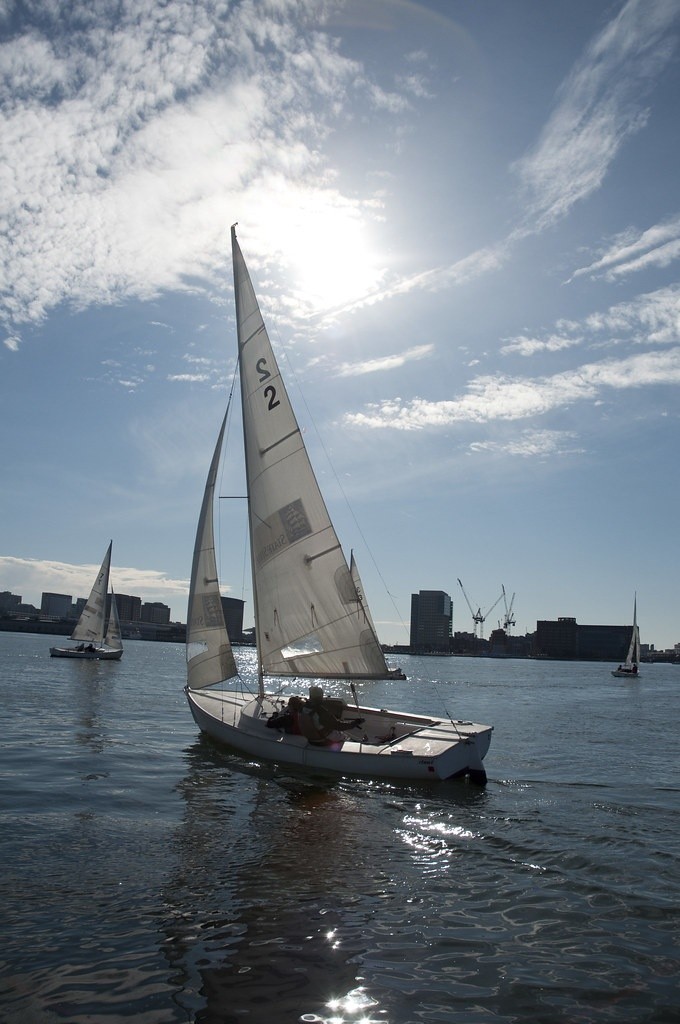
502,583,517,636
456,578,505,638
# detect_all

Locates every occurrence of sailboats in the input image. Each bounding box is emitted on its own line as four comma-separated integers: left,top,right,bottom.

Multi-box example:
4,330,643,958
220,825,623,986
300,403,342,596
610,590,641,677
181,221,493,785
49,539,124,659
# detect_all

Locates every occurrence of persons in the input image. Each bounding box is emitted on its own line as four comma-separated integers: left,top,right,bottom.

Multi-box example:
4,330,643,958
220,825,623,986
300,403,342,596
85,644,95,652
618,665,622,671
632,664,637,673
266,697,301,734
78,643,84,651
299,687,365,746
